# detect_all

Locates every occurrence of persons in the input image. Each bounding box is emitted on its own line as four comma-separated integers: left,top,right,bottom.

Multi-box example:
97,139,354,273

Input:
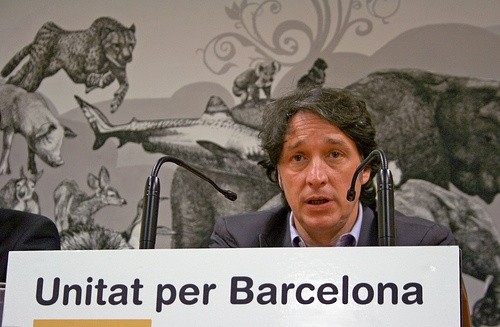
209,85,456,327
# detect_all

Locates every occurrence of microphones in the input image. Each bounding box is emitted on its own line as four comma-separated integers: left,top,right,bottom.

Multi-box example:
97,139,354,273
140,156,238,250
346,149,395,246
